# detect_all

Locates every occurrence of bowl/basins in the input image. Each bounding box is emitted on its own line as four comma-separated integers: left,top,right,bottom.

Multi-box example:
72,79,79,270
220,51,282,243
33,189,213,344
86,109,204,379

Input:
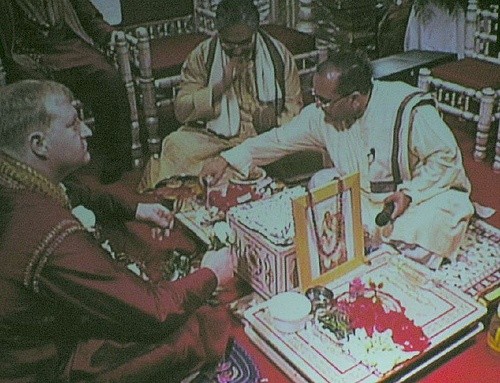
268,291,311,333
305,286,334,314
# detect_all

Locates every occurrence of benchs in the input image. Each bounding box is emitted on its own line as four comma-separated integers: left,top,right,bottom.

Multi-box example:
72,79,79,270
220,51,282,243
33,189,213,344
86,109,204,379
296,49,458,106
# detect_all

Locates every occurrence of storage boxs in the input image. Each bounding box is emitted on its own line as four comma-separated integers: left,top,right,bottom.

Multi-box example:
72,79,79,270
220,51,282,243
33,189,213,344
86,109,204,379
226,186,299,300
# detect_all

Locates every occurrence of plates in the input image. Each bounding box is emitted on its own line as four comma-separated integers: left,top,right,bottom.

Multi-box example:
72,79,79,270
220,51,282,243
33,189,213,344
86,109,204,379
315,308,350,344
229,167,266,184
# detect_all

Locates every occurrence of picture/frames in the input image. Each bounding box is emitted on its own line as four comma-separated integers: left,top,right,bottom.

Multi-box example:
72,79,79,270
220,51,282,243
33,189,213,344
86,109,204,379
291,171,365,292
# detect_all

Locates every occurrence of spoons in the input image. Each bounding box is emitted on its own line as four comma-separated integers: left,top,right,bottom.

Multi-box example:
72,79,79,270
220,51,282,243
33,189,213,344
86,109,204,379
159,197,183,236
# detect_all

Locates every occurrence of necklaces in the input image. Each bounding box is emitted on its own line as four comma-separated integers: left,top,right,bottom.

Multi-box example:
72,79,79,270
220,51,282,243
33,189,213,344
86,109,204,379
306,176,345,257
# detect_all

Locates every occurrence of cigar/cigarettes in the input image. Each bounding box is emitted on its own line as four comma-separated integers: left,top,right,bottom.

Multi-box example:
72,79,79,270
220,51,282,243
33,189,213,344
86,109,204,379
232,66,236,79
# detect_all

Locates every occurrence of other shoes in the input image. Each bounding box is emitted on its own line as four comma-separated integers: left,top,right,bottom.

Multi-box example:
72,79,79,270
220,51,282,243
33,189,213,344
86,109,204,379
99,146,134,184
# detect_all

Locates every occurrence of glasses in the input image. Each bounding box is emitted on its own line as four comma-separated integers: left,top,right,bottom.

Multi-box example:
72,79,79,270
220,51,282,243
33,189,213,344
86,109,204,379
312,88,358,112
220,35,252,51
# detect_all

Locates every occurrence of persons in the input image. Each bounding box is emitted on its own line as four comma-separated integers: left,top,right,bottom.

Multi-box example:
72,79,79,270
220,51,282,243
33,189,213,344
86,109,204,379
316,204,345,265
0,0,130,185
0,80,240,382
156,0,306,184
196,49,497,269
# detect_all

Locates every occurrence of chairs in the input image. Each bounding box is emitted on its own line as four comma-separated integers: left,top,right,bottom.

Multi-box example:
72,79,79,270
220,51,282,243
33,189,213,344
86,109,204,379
0,1,340,170
0,0,315,170
417,10,500,163
